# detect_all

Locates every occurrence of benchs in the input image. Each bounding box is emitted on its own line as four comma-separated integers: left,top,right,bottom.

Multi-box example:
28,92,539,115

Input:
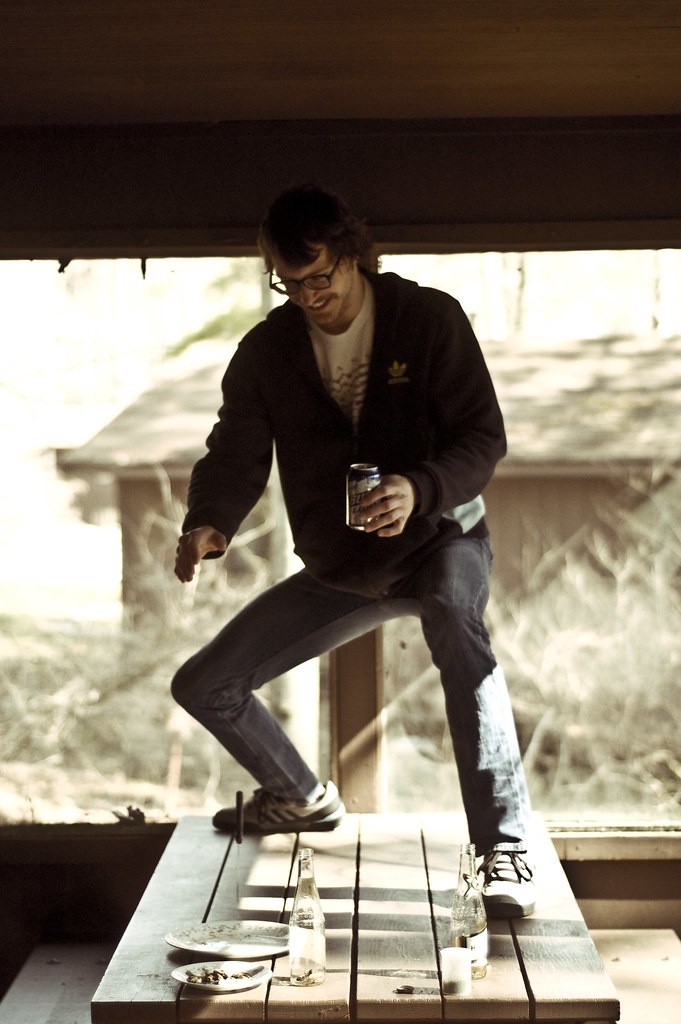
0,943,116,1024
590,928,681,1024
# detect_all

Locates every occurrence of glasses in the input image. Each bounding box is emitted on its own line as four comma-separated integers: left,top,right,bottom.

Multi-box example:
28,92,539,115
268,257,345,296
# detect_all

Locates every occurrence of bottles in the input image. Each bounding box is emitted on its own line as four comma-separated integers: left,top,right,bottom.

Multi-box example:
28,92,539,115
449,843,489,979
288,847,327,987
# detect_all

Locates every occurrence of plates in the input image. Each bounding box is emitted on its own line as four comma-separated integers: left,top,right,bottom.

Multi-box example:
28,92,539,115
171,961,273,992
165,920,290,959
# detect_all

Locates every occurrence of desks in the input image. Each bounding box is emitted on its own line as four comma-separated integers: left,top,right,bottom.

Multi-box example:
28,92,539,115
91,813,621,1024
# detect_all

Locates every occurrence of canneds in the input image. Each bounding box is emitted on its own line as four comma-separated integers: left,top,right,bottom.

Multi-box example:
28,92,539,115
346,464,382,530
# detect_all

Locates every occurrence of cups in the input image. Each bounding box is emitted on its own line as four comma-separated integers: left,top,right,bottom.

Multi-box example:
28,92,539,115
440,947,473,995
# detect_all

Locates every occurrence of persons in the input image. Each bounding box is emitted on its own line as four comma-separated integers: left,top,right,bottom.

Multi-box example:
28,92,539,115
171,185,532,918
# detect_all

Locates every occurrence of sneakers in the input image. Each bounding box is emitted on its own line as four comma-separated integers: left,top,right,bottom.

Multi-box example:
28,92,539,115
472,834,537,920
213,780,346,835
